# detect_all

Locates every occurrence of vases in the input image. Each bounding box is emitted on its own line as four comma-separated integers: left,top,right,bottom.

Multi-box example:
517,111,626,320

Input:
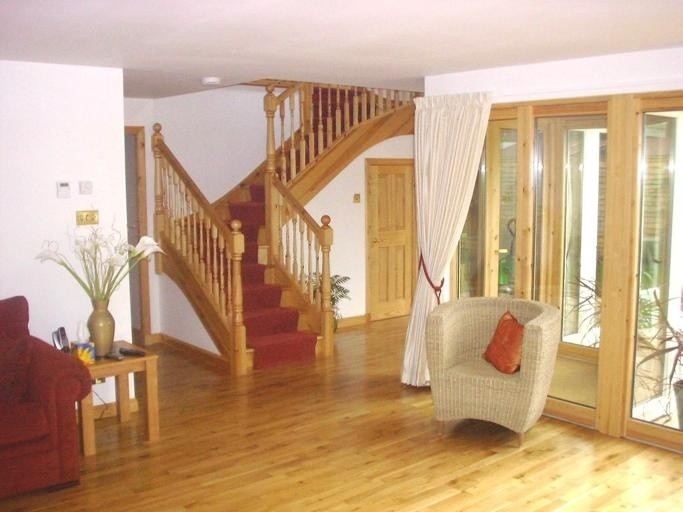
86,294,115,357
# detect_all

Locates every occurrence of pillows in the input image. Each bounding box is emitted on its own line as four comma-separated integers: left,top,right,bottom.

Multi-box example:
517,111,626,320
481,312,524,375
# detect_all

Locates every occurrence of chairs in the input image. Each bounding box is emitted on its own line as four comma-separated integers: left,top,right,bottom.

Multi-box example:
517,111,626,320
425,295,562,450
0,296,92,500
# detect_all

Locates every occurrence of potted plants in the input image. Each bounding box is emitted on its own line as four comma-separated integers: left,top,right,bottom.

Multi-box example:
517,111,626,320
306,271,350,332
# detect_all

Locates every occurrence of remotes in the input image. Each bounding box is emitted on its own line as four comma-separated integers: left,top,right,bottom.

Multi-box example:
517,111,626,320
107,353,124,361
119,347,145,356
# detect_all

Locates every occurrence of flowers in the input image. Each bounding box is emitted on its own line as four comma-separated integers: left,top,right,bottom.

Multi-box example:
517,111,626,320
33,228,169,302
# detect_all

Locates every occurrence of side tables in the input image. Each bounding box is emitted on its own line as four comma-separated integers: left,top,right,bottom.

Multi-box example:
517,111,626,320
76,341,160,457
